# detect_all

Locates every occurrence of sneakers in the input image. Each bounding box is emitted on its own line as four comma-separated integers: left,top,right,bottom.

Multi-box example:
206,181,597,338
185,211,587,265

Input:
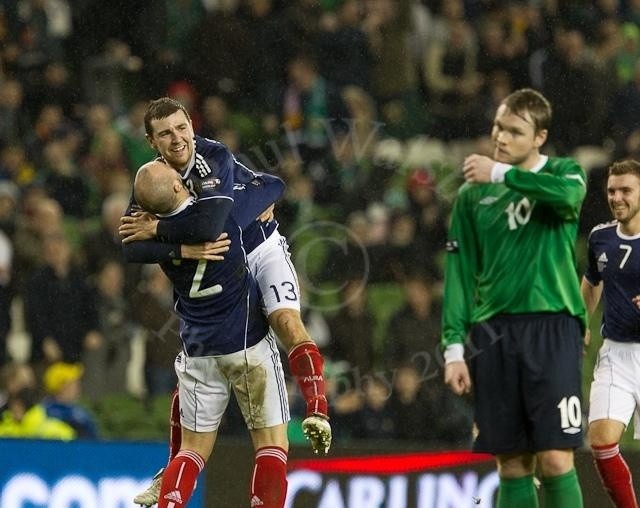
133,468,166,507
302,416,332,454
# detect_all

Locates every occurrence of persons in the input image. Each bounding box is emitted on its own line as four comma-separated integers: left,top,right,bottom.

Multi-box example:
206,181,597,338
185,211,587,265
118,97,332,508
0,1,640,446
133,162,292,508
579,158,640,508
440,88,590,508
